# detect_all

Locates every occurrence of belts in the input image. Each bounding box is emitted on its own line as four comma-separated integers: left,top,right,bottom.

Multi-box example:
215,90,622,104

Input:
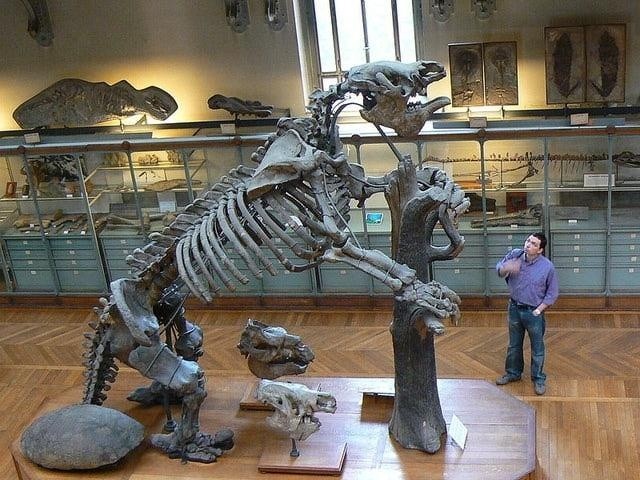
511,298,536,310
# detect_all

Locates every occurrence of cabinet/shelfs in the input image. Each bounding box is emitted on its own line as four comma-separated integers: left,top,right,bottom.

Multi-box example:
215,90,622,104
1,115,638,301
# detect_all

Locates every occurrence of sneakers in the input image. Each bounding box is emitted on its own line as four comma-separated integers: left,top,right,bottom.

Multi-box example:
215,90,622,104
496,373,520,384
534,379,546,395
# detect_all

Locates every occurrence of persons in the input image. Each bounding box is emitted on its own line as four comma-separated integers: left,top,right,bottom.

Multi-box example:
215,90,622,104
495,231,560,396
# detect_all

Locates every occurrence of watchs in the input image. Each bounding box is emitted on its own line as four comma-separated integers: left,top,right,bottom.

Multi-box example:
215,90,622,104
535,308,541,315
